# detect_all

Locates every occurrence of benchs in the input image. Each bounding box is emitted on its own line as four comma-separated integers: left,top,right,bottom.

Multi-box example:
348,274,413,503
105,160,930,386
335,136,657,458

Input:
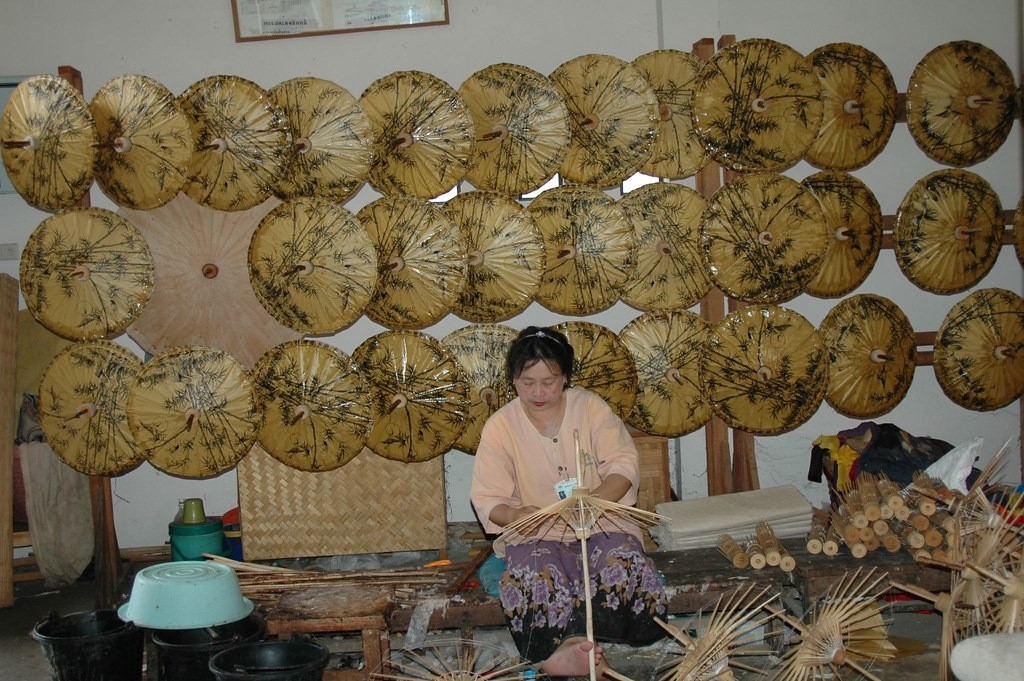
392,549,783,671
780,538,953,636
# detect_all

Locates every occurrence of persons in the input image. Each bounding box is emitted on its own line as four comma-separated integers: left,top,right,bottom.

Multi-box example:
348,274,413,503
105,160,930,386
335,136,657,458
468,326,667,678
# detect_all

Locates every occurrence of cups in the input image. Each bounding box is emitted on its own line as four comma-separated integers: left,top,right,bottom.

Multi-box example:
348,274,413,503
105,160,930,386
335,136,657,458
182,499,207,524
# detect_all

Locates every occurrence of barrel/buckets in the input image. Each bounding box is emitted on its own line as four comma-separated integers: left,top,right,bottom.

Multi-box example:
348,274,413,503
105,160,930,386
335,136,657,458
208,637,330,681
32,609,144,681
168,516,231,561
151,614,266,681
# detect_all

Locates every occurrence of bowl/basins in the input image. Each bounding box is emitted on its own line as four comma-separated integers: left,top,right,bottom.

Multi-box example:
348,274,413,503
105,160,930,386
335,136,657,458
116,562,254,629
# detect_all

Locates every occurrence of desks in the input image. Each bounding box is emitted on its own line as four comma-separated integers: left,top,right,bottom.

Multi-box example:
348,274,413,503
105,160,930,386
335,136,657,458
264,583,395,681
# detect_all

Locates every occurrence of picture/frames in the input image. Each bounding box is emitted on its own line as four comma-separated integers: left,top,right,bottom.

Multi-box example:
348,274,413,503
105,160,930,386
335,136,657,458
230,0,449,44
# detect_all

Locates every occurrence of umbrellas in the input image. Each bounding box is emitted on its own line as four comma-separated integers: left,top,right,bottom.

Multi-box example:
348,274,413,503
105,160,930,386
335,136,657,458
0,38,1023,680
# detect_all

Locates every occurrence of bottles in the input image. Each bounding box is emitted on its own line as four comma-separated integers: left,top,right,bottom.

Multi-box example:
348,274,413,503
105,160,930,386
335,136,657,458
173,498,185,523
